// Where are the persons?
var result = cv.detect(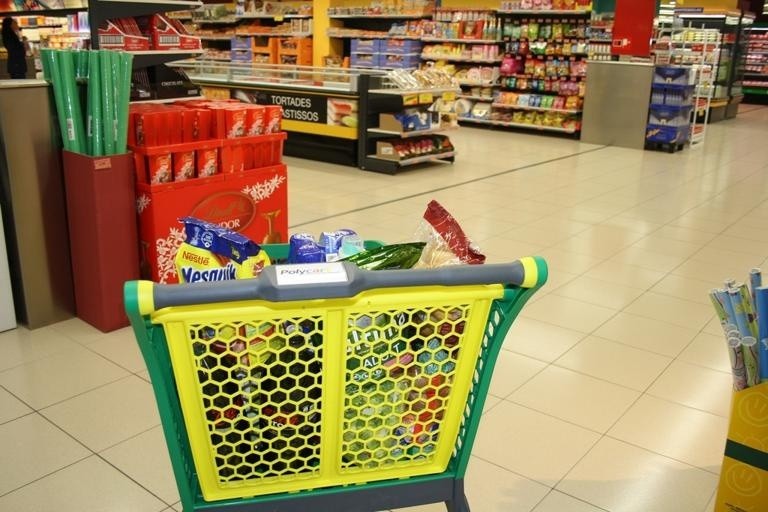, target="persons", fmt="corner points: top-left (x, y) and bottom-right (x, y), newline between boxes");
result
(1, 18), (28, 79)
